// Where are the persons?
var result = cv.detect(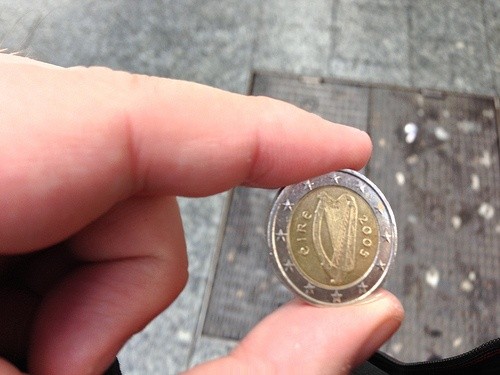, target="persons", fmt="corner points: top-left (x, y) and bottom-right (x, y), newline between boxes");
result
(0, 52), (499, 375)
